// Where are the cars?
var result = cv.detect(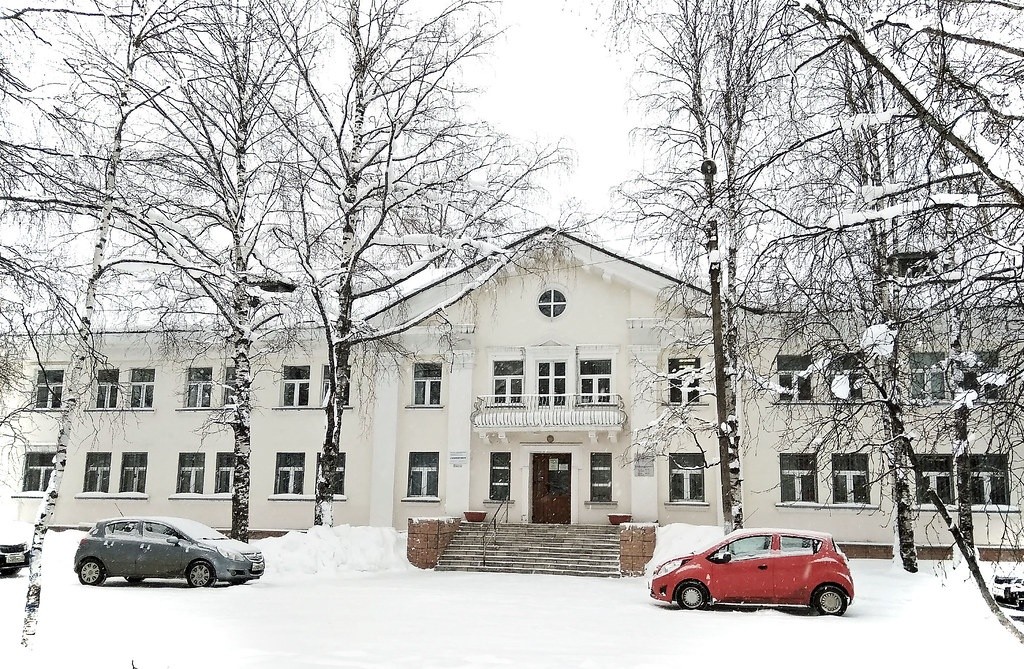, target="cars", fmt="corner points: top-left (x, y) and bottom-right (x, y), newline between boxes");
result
(645, 528), (854, 617)
(71, 514), (268, 591)
